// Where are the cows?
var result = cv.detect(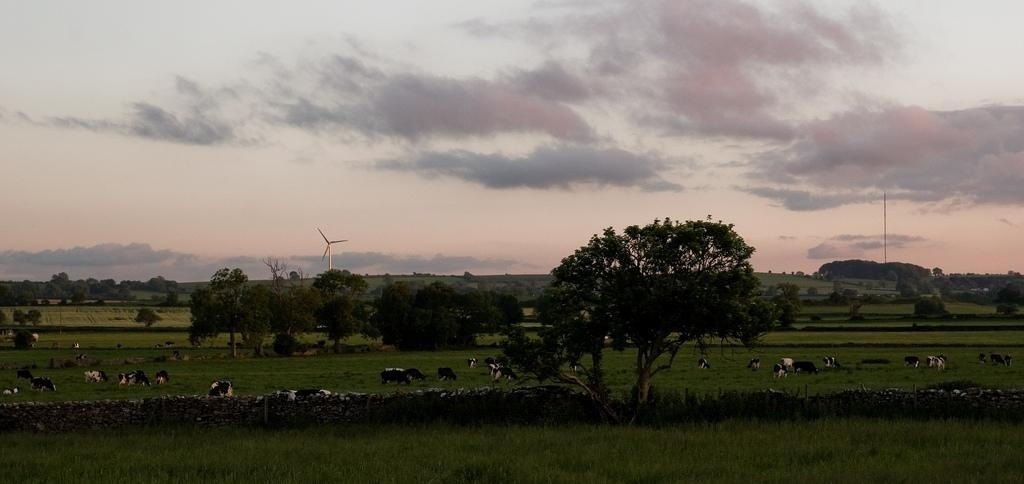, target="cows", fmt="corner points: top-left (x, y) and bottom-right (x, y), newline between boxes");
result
(3, 367), (59, 396)
(381, 357), (519, 385)
(903, 353), (947, 371)
(83, 369), (171, 387)
(748, 357), (761, 370)
(978, 352), (1014, 368)
(698, 357), (710, 369)
(210, 378), (236, 400)
(772, 356), (820, 379)
(822, 355), (838, 369)
